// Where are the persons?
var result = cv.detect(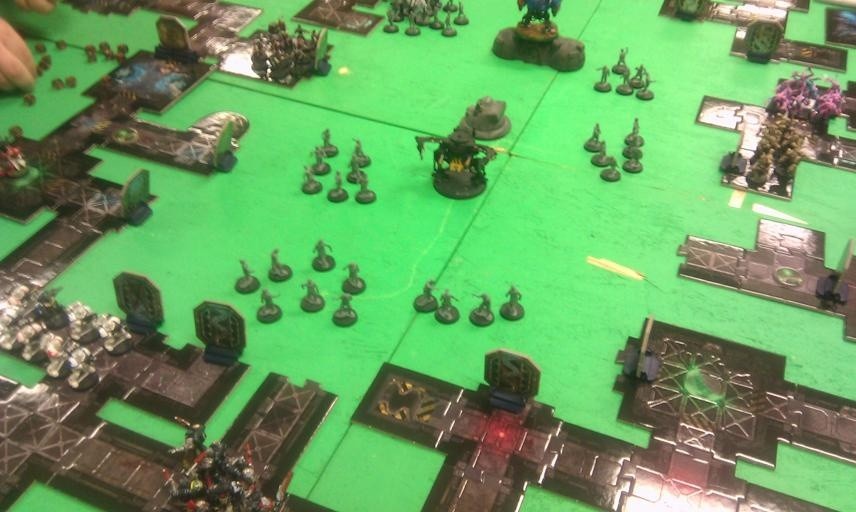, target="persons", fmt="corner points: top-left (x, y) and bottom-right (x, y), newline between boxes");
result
(335, 293), (352, 315)
(247, 19), (329, 81)
(590, 47), (657, 179)
(382, 0), (468, 36)
(473, 292), (491, 317)
(260, 287), (281, 313)
(342, 262), (360, 285)
(312, 240), (333, 265)
(0, 0), (56, 93)
(271, 248), (283, 275)
(162, 412), (292, 512)
(419, 280), (436, 303)
(300, 279), (320, 302)
(506, 285), (521, 309)
(748, 70), (844, 190)
(239, 259), (253, 280)
(441, 288), (459, 318)
(302, 127), (372, 198)
